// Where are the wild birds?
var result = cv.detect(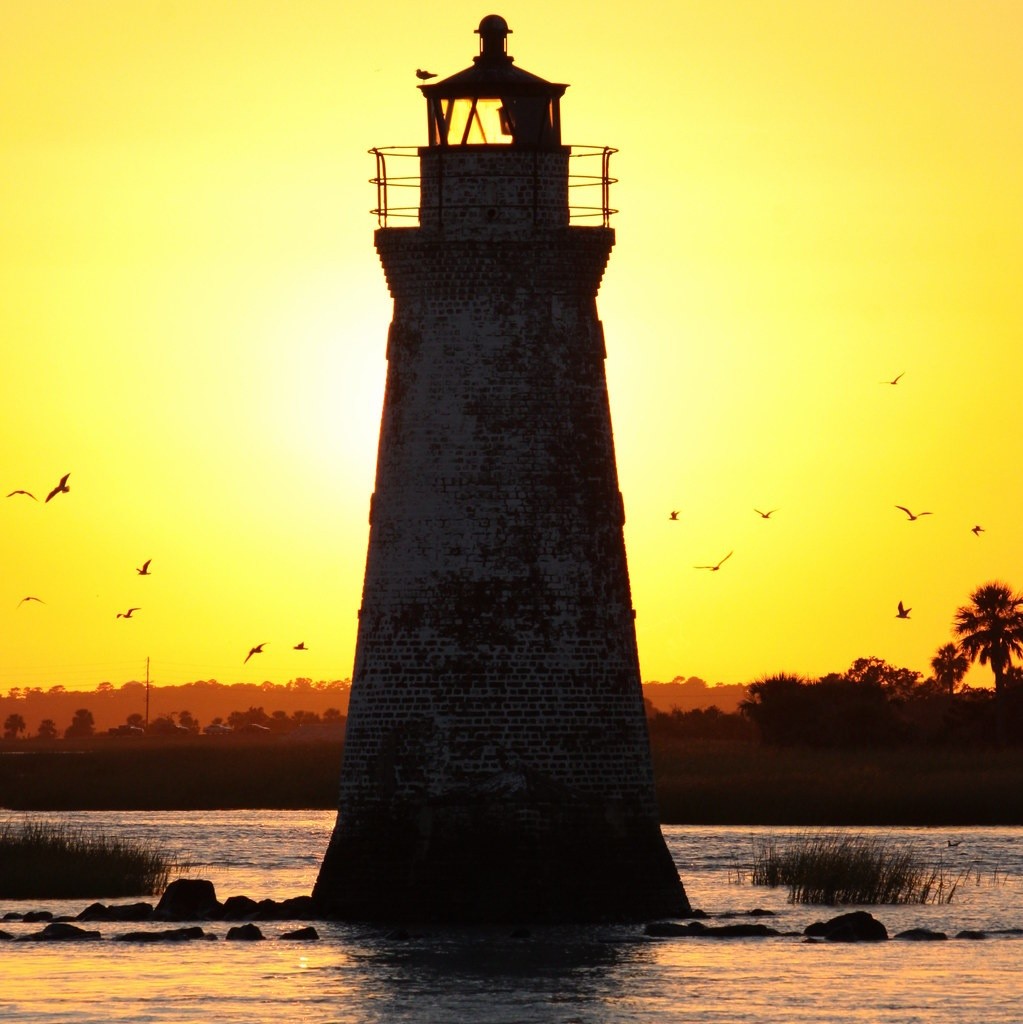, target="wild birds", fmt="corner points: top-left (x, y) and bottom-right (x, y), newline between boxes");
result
(46, 473), (70, 502)
(243, 643), (266, 665)
(695, 552), (732, 571)
(17, 597), (44, 607)
(754, 509), (776, 518)
(7, 491), (36, 500)
(971, 525), (984, 535)
(669, 511), (679, 520)
(416, 69), (437, 83)
(294, 642), (307, 649)
(896, 601), (912, 618)
(117, 608), (141, 618)
(897, 505), (932, 520)
(947, 840), (961, 846)
(882, 373), (903, 384)
(136, 560), (151, 574)
